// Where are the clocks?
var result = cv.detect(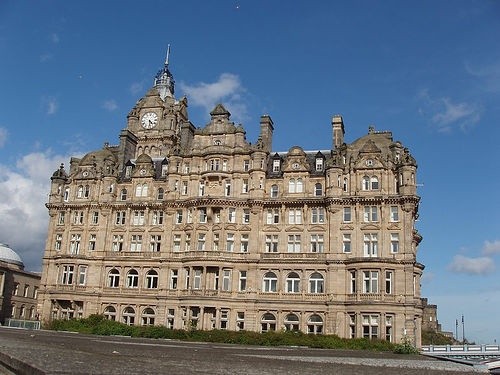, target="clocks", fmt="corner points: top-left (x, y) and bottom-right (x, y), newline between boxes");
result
(140, 111), (159, 130)
(291, 161), (299, 172)
(139, 168), (147, 177)
